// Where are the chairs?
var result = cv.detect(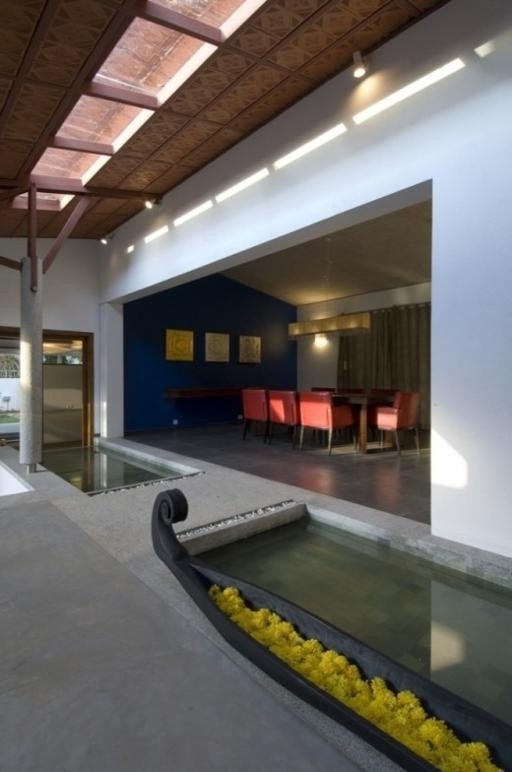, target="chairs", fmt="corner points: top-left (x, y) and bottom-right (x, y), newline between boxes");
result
(237, 385), (424, 459)
